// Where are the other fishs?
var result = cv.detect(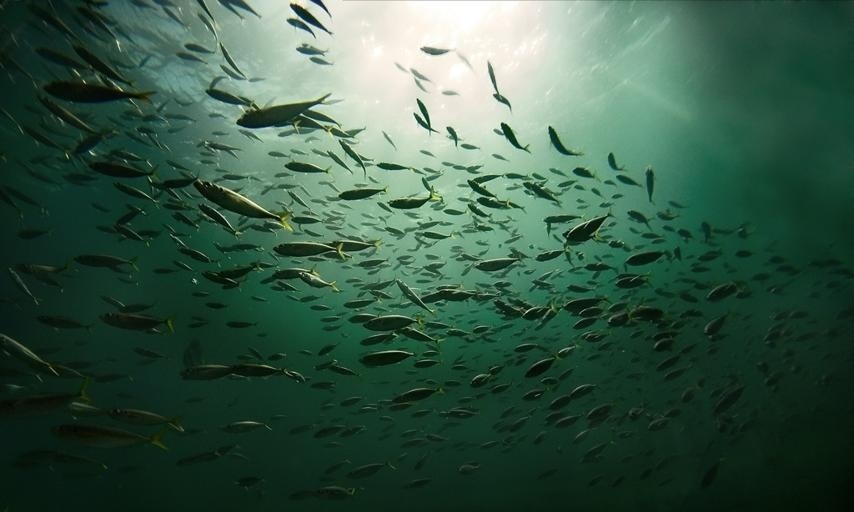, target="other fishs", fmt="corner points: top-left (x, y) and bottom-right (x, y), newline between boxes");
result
(0, 0), (854, 511)
